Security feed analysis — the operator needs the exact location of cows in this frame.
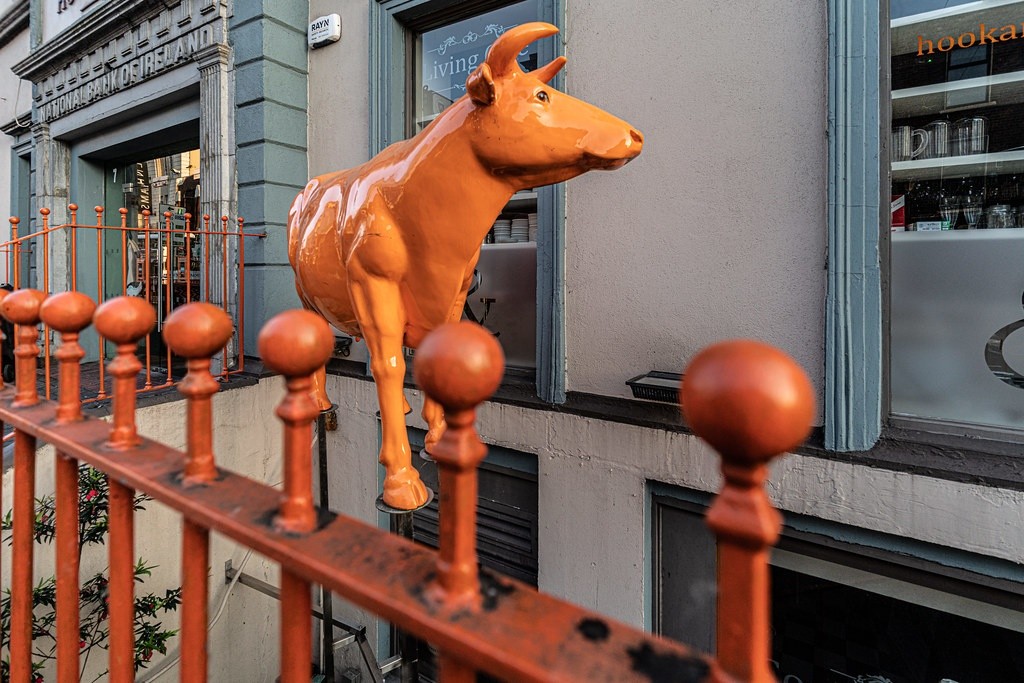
[287,22,645,512]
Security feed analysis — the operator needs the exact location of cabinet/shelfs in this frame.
[417,112,537,250]
[890,0,1024,240]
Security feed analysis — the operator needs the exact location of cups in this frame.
[890,115,985,160]
[899,170,1018,231]
